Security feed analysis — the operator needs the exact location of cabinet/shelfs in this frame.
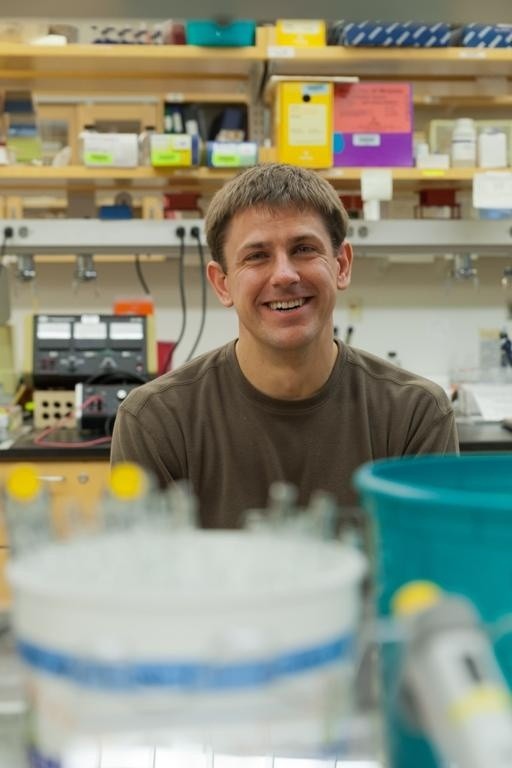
[0,39,511,458]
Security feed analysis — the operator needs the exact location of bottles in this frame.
[479,126,507,167]
[390,579,511,768]
[451,116,476,167]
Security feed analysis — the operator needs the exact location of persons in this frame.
[109,162,460,593]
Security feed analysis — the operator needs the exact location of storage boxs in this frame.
[268,81,335,170]
[78,118,144,167]
[149,133,192,169]
[205,139,259,170]
[184,17,255,44]
[275,17,327,44]
[332,81,414,168]
